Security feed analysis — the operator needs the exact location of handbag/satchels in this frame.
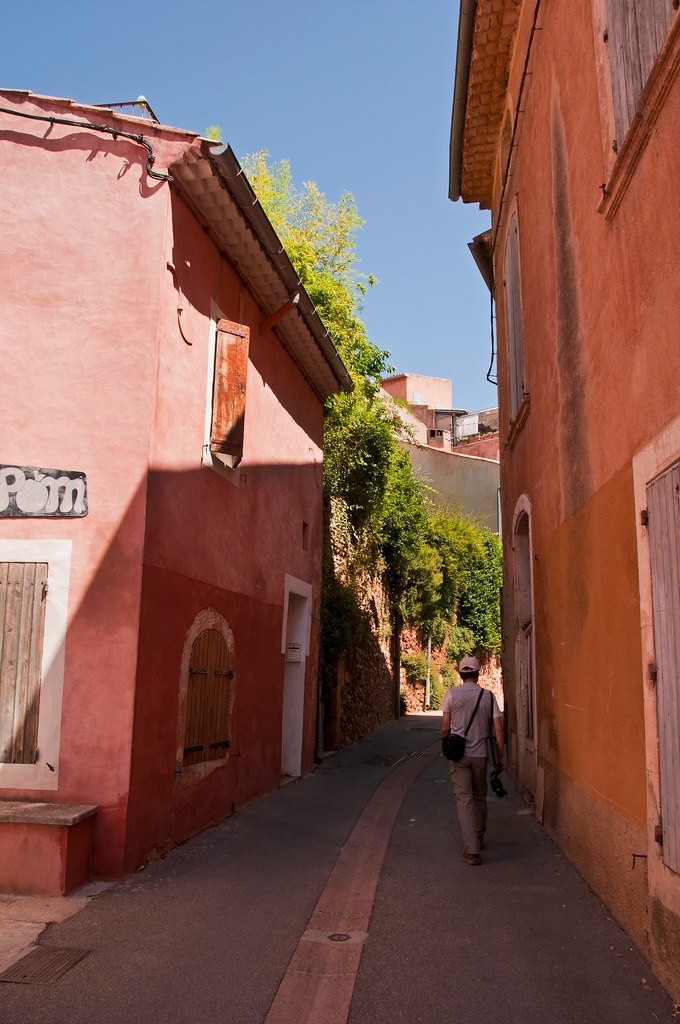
[486,737,499,765]
[441,734,466,760]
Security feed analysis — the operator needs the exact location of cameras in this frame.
[491,779,508,799]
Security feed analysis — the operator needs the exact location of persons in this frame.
[440,656,505,865]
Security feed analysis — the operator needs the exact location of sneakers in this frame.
[462,852,481,865]
[479,838,484,849]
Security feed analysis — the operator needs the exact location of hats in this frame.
[458,657,479,673]
